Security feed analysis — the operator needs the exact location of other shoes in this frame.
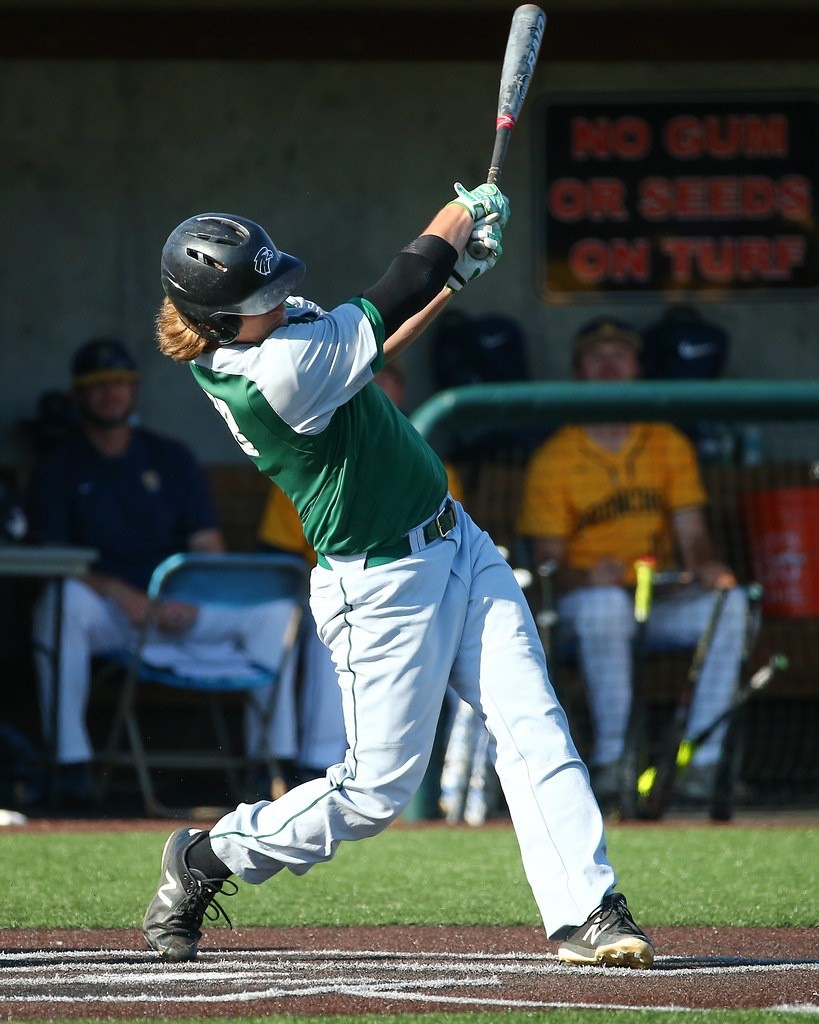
[594,759,636,793]
[609,745,714,758]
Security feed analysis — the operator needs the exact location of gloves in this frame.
[446,181,511,231]
[444,212,503,295]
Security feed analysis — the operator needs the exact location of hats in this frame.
[568,315,641,355]
[70,338,140,387]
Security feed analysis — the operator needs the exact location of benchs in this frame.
[203,464,818,696]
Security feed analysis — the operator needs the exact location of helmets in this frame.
[161,213,307,345]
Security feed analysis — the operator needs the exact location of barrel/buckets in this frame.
[741,488,818,618]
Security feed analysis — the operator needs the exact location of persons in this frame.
[260,362,464,775]
[514,313,747,798]
[22,338,298,815]
[141,183,654,968]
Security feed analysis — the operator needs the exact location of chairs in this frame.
[525,568,762,804]
[88,550,311,818]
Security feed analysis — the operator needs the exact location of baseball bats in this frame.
[464,3,549,263]
[436,548,795,824]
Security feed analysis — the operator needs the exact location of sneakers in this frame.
[140,827,239,962]
[558,892,655,969]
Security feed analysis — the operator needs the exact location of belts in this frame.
[317,500,458,570]
